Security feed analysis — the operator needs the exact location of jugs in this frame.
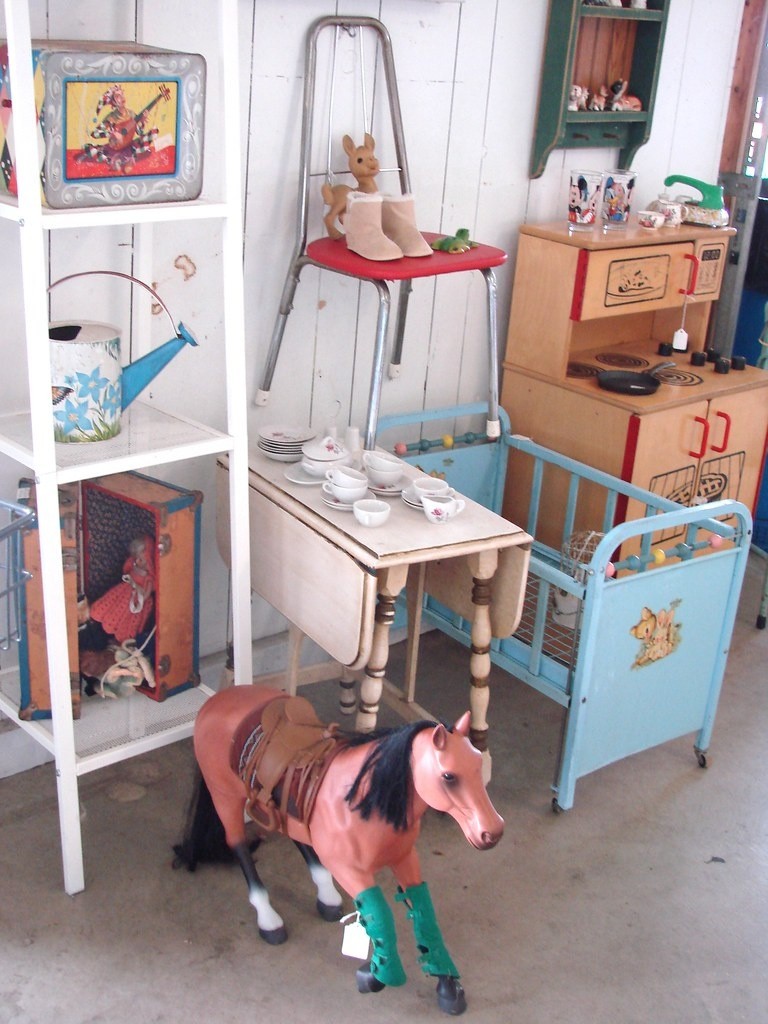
[46,268,201,446]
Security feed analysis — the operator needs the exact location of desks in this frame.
[216,436,533,789]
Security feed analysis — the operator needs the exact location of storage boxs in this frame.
[14,471,204,723]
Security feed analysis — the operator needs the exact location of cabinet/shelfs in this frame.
[0,1,275,896]
[528,1,671,179]
[500,220,767,579]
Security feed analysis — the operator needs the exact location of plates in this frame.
[256,424,316,462]
[284,461,331,486]
[319,490,377,512]
[369,476,413,497]
[400,487,424,511]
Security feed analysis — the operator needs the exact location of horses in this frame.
[168,683,504,1017]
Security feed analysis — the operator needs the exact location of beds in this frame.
[372,401,753,817]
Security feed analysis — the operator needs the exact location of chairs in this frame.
[255,14,508,450]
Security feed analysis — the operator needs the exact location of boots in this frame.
[381,194,434,257]
[345,192,403,260]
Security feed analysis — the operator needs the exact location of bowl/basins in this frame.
[636,210,665,231]
[301,436,353,478]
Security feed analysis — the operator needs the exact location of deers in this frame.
[320,133,383,243]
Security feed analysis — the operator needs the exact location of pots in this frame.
[597,361,677,395]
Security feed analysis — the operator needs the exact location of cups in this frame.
[657,199,689,229]
[412,477,449,503]
[567,169,606,232]
[363,448,404,485]
[603,169,638,230]
[420,494,466,524]
[352,499,391,528]
[322,465,369,504]
